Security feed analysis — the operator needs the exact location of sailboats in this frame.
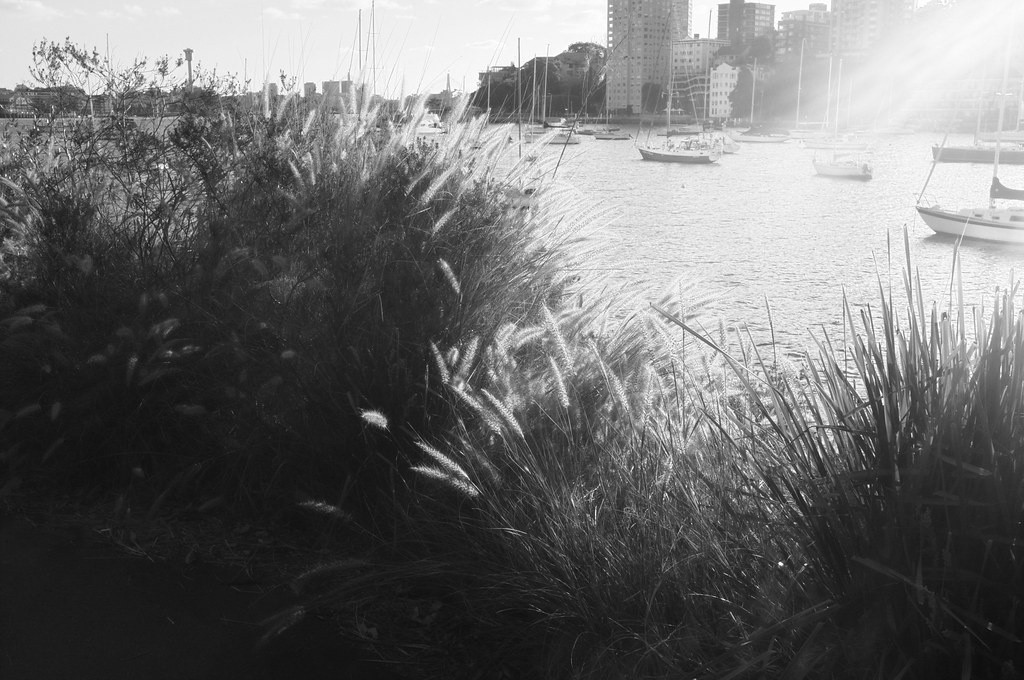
[261,0,1024,246]
